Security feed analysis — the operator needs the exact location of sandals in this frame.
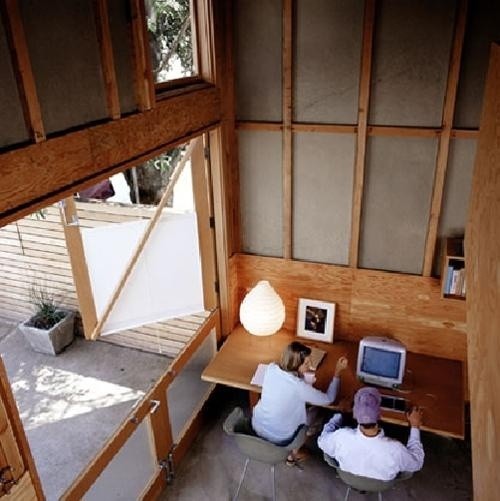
[286,451,309,466]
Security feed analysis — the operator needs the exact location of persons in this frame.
[251,341,348,467]
[318,387,425,493]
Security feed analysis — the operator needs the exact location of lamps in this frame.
[239,280,286,337]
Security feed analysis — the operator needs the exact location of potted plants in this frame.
[18,284,76,358]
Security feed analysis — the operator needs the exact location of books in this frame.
[444,266,466,298]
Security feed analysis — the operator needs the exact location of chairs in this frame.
[323,452,414,501]
[222,406,308,501]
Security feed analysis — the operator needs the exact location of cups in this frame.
[303,372,317,386]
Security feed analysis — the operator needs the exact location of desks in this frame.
[201,322,465,441]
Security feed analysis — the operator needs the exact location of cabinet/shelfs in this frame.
[439,236,466,304]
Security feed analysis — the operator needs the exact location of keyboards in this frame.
[379,394,407,414]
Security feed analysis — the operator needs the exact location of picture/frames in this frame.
[296,298,336,345]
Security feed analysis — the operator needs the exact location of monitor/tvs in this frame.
[356,336,406,390]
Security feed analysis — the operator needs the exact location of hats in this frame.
[352,388,381,424]
